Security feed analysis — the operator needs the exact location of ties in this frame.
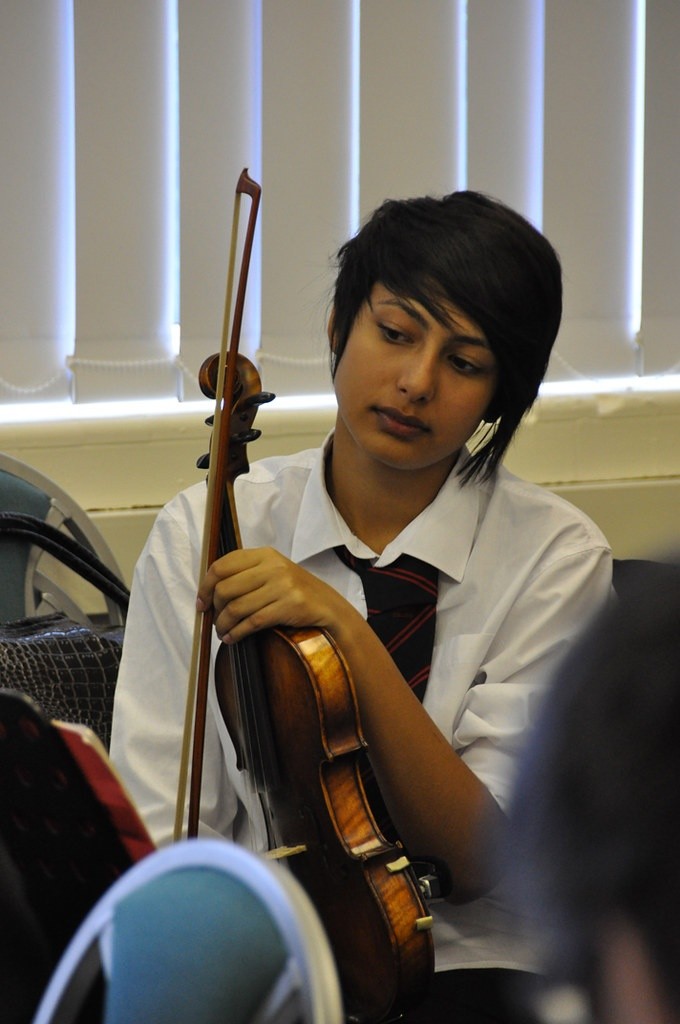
[335,546,438,848]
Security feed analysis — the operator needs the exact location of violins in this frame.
[196,352,435,1024]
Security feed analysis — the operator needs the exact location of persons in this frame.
[461,542,680,1024]
[109,191,612,1024]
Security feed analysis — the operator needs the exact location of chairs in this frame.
[0,454,344,1024]
